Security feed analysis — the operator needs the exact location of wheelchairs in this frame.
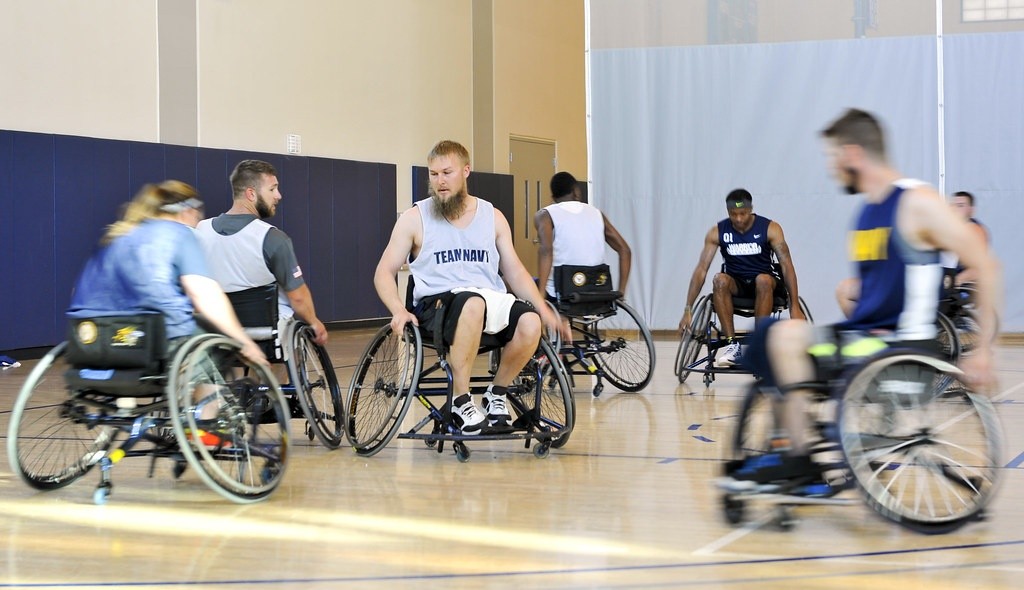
[675,263,815,389]
[343,273,577,465]
[6,310,291,505]
[478,264,657,397]
[189,282,345,449]
[935,278,1003,354]
[722,325,1008,535]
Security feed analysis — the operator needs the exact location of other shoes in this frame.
[182,420,233,448]
[234,395,271,406]
[284,394,299,400]
[537,355,563,364]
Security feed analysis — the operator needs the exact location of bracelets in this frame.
[685,306,691,312]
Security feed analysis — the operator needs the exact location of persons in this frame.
[501,172,631,308]
[374,141,573,434]
[680,189,805,366]
[68,181,271,450]
[196,160,328,411]
[837,193,989,335]
[714,110,1000,499]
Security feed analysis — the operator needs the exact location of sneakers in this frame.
[451,392,492,436]
[480,383,512,426]
[715,342,742,366]
[714,453,833,495]
